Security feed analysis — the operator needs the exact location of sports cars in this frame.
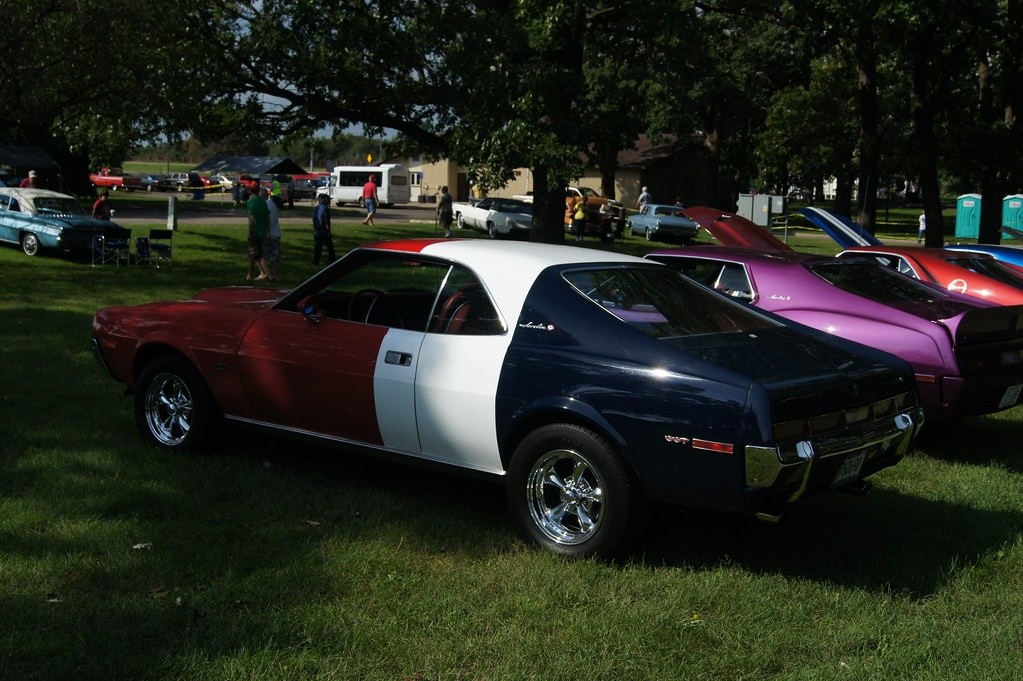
[588,206,1023,436]
[87,236,925,566]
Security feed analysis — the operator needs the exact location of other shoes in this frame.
[255,274,267,281]
[363,222,369,225]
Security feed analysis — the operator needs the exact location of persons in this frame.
[92,189,111,222]
[436,186,454,238]
[20,170,40,188]
[890,180,918,198]
[313,193,336,264]
[918,210,926,244]
[233,176,294,280]
[636,186,652,208]
[362,173,379,227]
[572,195,589,243]
[598,198,614,243]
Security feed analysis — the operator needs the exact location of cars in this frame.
[163,171,326,203]
[133,173,172,190]
[0,186,128,259]
[625,203,699,243]
[449,196,537,238]
[88,167,142,192]
[514,187,626,236]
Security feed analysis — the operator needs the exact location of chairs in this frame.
[366,287,433,329]
[108,228,132,268]
[91,235,118,269]
[144,229,173,266]
[134,236,154,268]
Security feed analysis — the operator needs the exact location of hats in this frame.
[322,194,333,201]
[369,175,376,181]
[29,170,37,177]
[249,181,260,191]
[260,188,271,198]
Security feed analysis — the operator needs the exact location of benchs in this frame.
[431,286,494,334]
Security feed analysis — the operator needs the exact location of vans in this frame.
[315,164,413,208]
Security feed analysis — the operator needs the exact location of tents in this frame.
[190,155,310,176]
